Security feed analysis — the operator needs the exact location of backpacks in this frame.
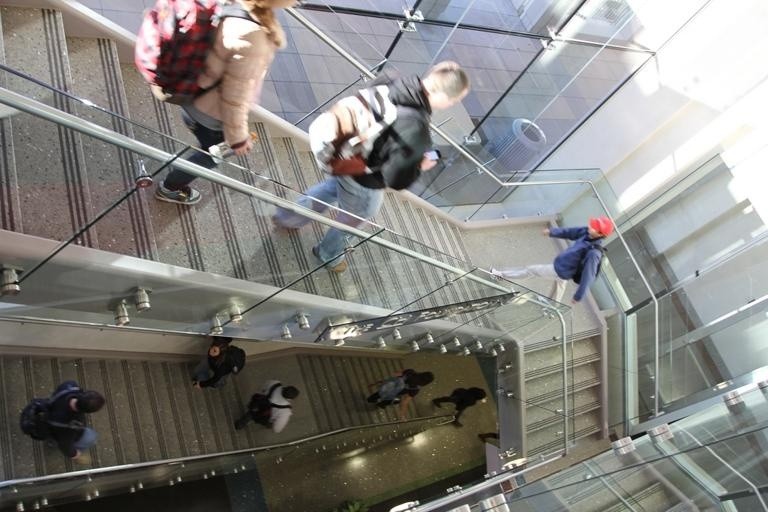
[572,244,608,285]
[307,80,429,177]
[378,371,420,401]
[216,345,246,375]
[248,383,291,425]
[20,385,85,440]
[133,0,262,105]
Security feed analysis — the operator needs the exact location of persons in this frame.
[133,1,295,205]
[189,335,245,389]
[432,386,487,428]
[366,368,434,418]
[21,381,106,459]
[234,379,302,434]
[267,60,470,276]
[489,217,615,310]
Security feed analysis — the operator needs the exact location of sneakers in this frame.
[312,243,349,273]
[432,398,442,408]
[451,420,463,428]
[490,267,505,281]
[154,180,203,205]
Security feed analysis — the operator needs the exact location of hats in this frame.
[589,213,614,239]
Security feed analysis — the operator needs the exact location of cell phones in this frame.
[423,149,442,161]
